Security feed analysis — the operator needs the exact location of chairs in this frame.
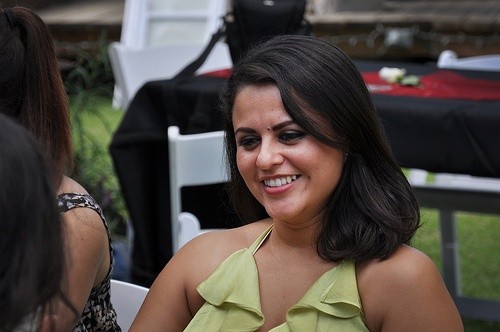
[167,126,255,264]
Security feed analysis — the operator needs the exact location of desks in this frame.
[110,56,500,312]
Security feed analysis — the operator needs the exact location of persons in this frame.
[1,115,69,331]
[124,35,466,332]
[1,8,119,331]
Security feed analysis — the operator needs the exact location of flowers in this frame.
[378,67,425,90]
